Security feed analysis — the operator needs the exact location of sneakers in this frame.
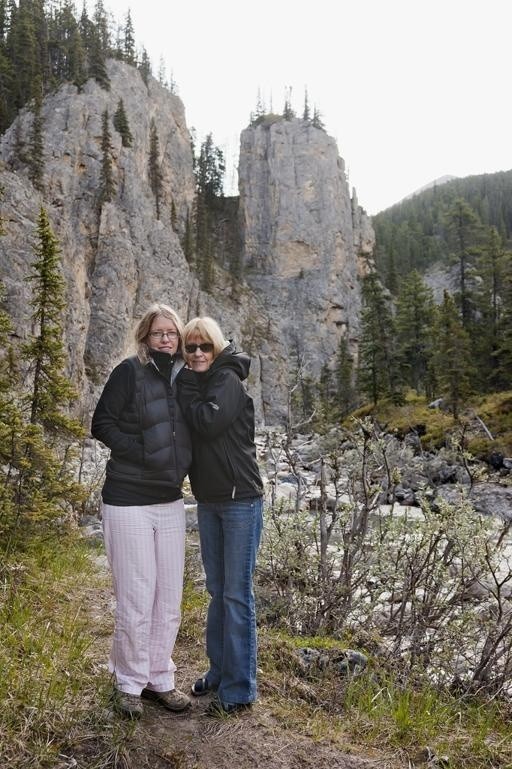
[110,687,142,719]
[191,671,252,718]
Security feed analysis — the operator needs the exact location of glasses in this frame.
[149,330,177,339]
[185,343,214,353]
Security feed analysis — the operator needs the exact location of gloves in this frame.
[143,686,192,713]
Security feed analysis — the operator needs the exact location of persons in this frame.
[91,303,193,715]
[176,316,266,716]
